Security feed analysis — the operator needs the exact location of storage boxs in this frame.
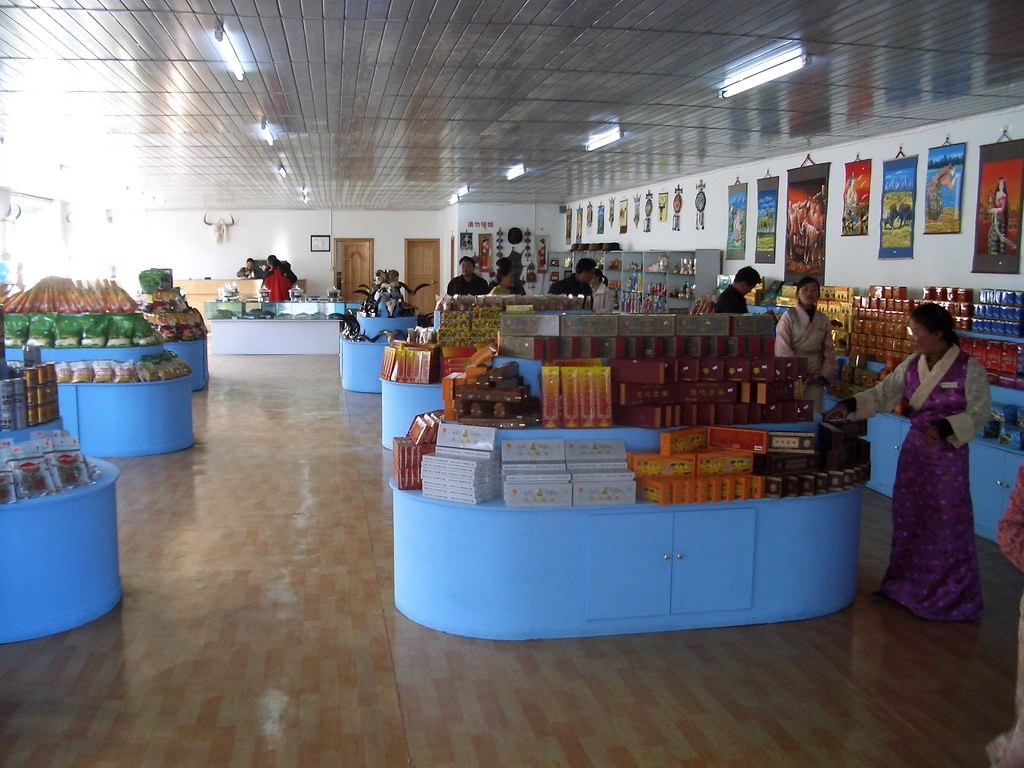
[380,259,1024,506]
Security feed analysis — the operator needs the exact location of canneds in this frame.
[0,345,59,432]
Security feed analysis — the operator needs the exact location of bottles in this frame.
[620,258,669,313]
[671,257,696,300]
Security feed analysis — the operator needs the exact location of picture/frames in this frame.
[311,235,330,252]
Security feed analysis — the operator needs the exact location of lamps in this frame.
[214,31,244,82]
[302,194,308,205]
[449,196,460,205]
[718,53,814,99]
[261,122,274,147]
[278,166,287,179]
[507,168,528,182]
[585,132,625,152]
[459,187,470,197]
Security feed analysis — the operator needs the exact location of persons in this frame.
[261,255,297,285]
[447,256,490,295]
[489,258,526,296]
[237,258,264,279]
[261,262,291,303]
[488,268,516,295]
[774,275,837,413]
[822,302,992,622]
[712,266,761,313]
[589,269,613,313]
[547,257,596,309]
[987,462,1024,768]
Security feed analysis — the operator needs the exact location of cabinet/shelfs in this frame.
[0,416,121,644]
[377,310,446,451]
[137,308,210,391]
[389,358,862,641]
[747,304,1024,544]
[337,312,417,395]
[4,312,195,459]
[544,249,721,314]
